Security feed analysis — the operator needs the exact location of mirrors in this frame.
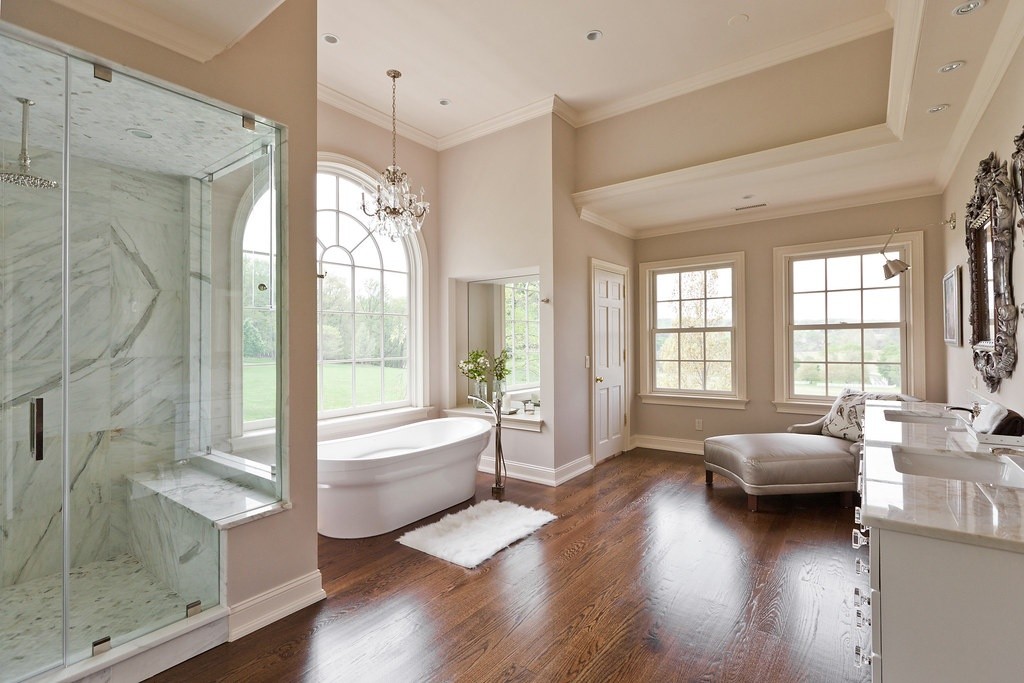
[963,151,1019,394]
[466,272,541,412]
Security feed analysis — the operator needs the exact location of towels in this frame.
[971,401,1024,437]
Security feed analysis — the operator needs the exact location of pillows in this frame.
[821,386,909,442]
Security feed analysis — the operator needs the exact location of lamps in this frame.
[880,211,957,280]
[360,166,430,242]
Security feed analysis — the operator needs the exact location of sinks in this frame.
[883,408,968,427]
[890,443,1024,489]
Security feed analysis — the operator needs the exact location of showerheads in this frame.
[1,96,59,191]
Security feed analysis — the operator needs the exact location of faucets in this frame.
[467,393,500,425]
[943,402,982,426]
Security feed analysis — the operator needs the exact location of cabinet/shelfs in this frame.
[852,447,1022,682]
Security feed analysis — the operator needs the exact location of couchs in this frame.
[701,388,925,511]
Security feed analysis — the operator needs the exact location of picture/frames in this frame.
[942,265,962,347]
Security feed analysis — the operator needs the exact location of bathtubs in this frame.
[317,416,493,539]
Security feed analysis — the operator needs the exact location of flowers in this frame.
[490,349,514,380]
[459,349,490,379]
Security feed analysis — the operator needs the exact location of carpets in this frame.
[394,498,558,570]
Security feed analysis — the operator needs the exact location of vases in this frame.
[472,382,487,408]
[492,380,508,411]
